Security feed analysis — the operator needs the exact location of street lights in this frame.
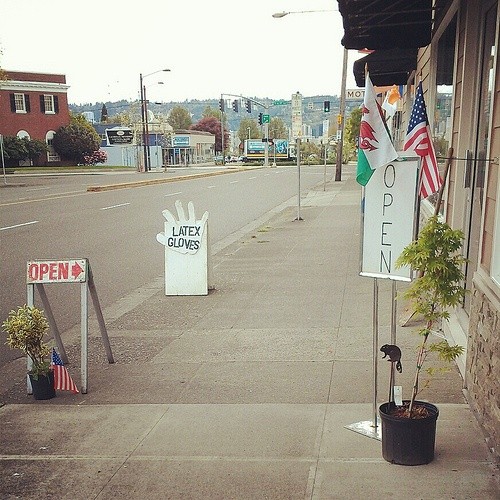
[140,69,172,172]
[143,81,165,171]
[272,9,349,182]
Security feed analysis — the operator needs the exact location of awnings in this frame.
[337,0,432,87]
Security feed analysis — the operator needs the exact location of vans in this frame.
[214,156,245,162]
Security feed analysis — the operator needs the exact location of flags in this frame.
[403,82,443,201]
[356,70,398,186]
[52,348,79,395]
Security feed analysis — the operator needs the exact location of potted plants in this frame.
[379,214,474,466]
[2,303,55,400]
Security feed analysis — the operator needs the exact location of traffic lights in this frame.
[219,99,225,112]
[245,100,251,113]
[324,101,330,112]
[232,99,238,113]
[258,113,263,126]
